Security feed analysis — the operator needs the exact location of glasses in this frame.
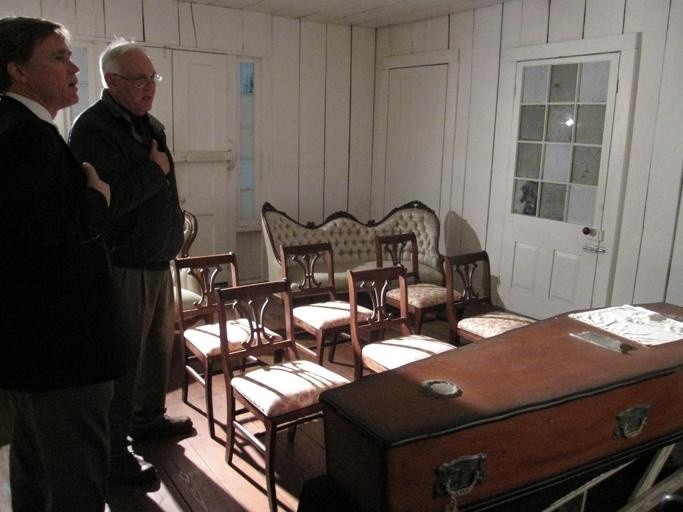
[112,73,158,90]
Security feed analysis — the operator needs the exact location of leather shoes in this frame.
[132,410,193,443]
[110,446,158,489]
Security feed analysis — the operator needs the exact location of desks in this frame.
[320,303,683,512]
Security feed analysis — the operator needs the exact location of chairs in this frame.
[172,251,285,439]
[374,234,466,335]
[279,241,379,368]
[436,249,537,349]
[346,263,458,382]
[214,277,351,511]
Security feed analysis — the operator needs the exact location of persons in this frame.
[1,13,130,512]
[58,30,195,484]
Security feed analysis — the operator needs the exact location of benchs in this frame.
[261,202,445,304]
[170,209,214,331]
